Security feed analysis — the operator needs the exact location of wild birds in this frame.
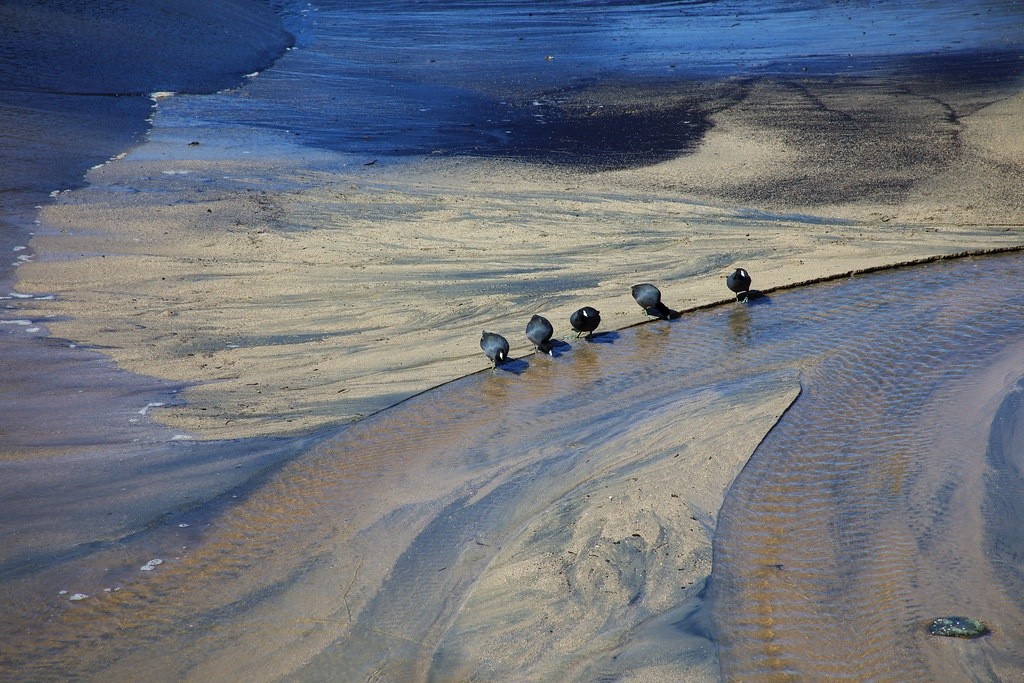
[726,267,752,304]
[630,283,672,321]
[479,329,510,372]
[569,306,601,343]
[525,314,554,357]
